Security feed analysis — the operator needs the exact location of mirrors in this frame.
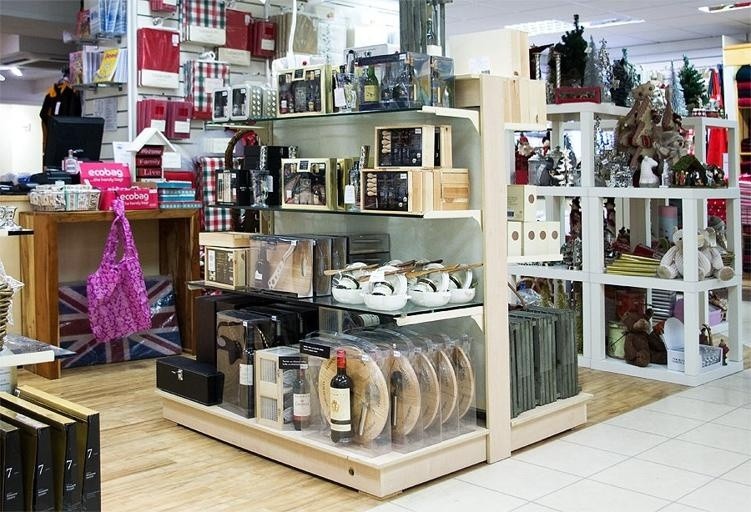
[1,0,138,189]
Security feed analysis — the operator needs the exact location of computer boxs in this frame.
[30,172,80,185]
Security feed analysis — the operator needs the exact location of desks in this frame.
[0,350,55,369]
[18,211,198,379]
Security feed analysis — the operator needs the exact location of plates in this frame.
[318,345,475,446]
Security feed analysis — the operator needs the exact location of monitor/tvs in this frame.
[44,116,105,169]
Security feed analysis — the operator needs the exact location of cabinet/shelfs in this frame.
[505,104,744,386]
[155,72,498,499]
[498,72,593,463]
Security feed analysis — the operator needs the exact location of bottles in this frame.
[255,247,270,288]
[292,369,310,431]
[280,83,321,114]
[64,149,78,175]
[363,62,441,109]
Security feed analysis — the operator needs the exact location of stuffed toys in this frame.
[606,288,684,367]
[656,215,735,281]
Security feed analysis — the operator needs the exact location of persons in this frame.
[602,198,631,267]
[515,130,582,188]
[699,328,708,344]
[559,197,582,270]
[719,339,729,365]
